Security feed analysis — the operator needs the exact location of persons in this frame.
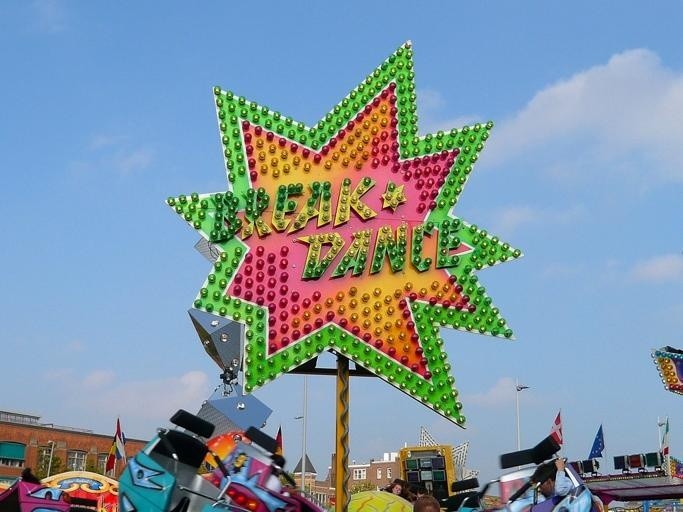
[410,488,428,506]
[521,459,576,512]
[390,483,403,495]
[413,494,440,512]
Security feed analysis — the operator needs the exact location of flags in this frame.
[114,419,125,460]
[587,424,604,459]
[551,412,562,445]
[657,416,671,448]
[105,438,116,474]
